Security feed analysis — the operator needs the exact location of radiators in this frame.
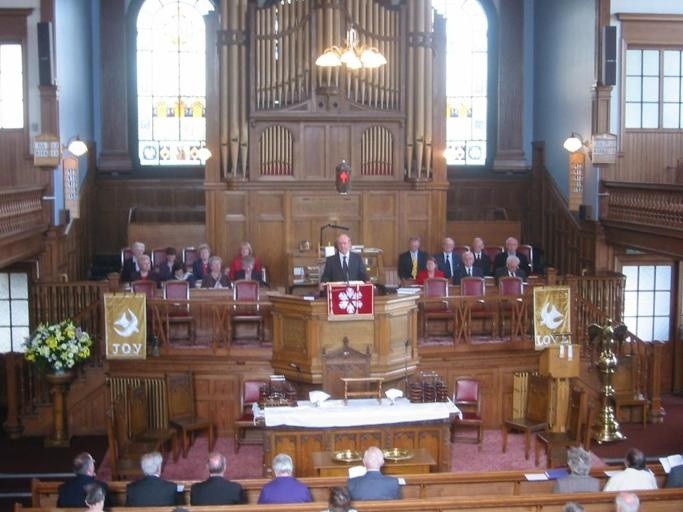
[104,376,168,438]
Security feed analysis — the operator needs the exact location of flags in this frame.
[533,286,573,351]
[326,283,375,321]
[103,290,148,362]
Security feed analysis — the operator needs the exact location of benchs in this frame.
[12,460,682,511]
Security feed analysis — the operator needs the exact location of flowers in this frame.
[19,318,94,371]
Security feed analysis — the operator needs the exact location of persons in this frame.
[319,233,374,293]
[322,484,358,512]
[233,255,264,283]
[56,450,111,509]
[452,250,485,283]
[226,240,264,279]
[493,235,529,275]
[470,235,492,276]
[397,236,429,282]
[603,444,660,492]
[168,258,197,287]
[415,255,446,284]
[125,449,183,508]
[613,491,644,512]
[493,253,528,286]
[551,445,602,493]
[119,241,154,279]
[256,452,315,505]
[188,449,243,507]
[82,481,108,512]
[127,254,161,286]
[200,254,230,288]
[192,242,213,277]
[346,444,403,500]
[154,246,187,278]
[434,235,463,278]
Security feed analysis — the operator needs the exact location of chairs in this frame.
[159,279,196,347]
[606,352,651,433]
[150,248,168,272]
[459,276,501,344]
[228,278,264,344]
[501,373,548,461]
[119,246,134,269]
[449,376,484,444]
[338,376,385,399]
[420,277,457,342]
[451,244,472,257]
[129,279,157,321]
[515,244,534,275]
[482,244,505,273]
[496,275,524,313]
[164,368,214,459]
[231,375,268,455]
[181,245,200,267]
[533,383,587,470]
[124,380,179,460]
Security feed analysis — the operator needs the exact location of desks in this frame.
[536,343,582,433]
[249,395,462,477]
[264,292,421,384]
[286,245,386,292]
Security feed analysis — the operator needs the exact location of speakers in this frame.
[38,22,54,86]
[601,26,616,85]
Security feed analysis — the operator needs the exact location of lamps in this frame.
[313,25,389,72]
[560,129,591,154]
[61,131,90,158]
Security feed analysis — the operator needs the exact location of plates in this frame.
[381,448,415,464]
[330,449,364,463]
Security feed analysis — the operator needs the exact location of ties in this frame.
[342,256,348,271]
[510,272,515,278]
[476,255,481,263]
[468,269,471,277]
[411,255,418,279]
[444,255,452,278]
